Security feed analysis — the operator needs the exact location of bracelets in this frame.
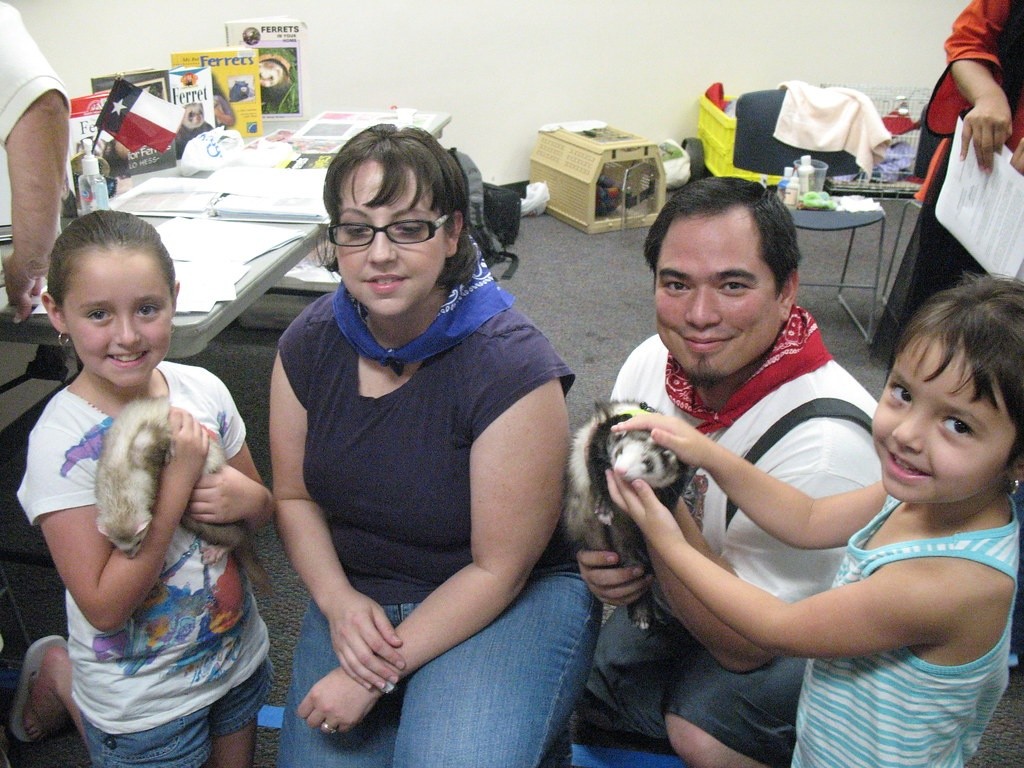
[380,681,395,693]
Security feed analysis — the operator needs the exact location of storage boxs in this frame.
[697,93,783,186]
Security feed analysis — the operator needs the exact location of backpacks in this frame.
[446,146,519,280]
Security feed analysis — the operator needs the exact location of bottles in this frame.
[798,155,816,194]
[783,176,800,206]
[776,167,794,201]
[78,139,109,215]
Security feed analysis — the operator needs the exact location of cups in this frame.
[794,159,829,194]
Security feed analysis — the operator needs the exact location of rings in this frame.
[321,722,336,733]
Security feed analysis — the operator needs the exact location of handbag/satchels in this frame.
[175,126,244,177]
[481,181,521,247]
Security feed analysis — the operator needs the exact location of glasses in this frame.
[329,215,451,247]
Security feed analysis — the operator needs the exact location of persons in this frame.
[267,124,605,768]
[12,211,273,768]
[1,0,72,324]
[580,0,1024,768]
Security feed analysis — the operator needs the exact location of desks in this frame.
[785,195,886,343]
[0,109,451,393]
[882,199,923,305]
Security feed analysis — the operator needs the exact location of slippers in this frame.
[9,635,71,743]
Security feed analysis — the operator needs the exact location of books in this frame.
[68,20,418,225]
[935,115,1023,281]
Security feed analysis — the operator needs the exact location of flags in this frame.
[96,72,185,154]
[103,130,145,153]
